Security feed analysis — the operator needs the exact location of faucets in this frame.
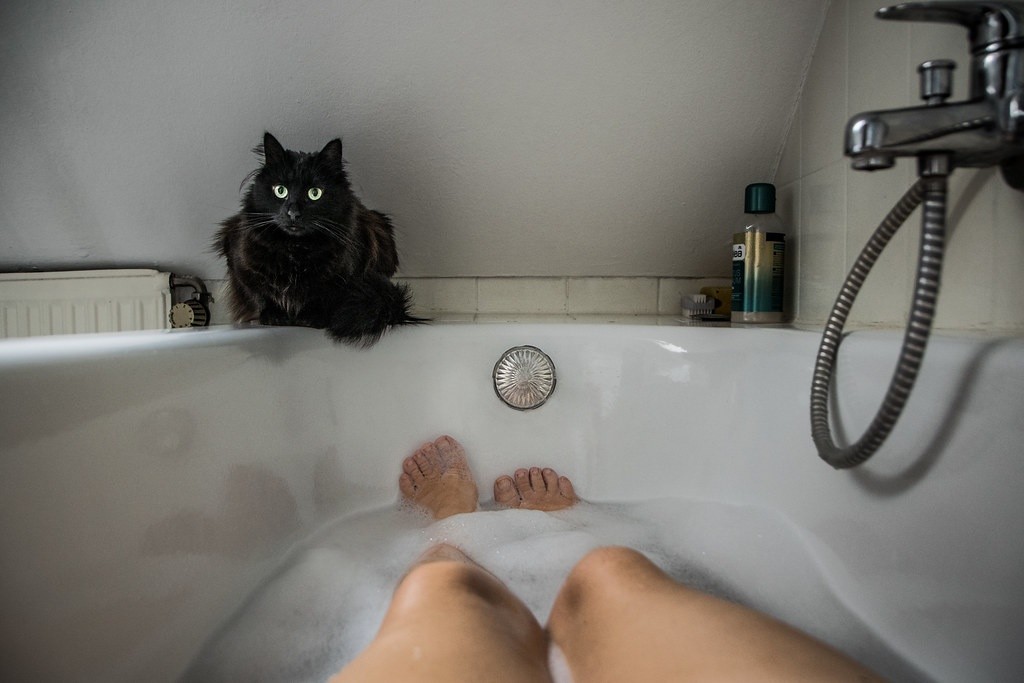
[842,0,1023,179]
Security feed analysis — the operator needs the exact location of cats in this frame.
[193,129,436,350]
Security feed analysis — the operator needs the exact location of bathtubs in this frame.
[0,321,1022,682]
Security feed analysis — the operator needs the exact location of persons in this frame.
[329,434,896,683]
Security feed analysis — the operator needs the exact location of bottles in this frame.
[730,182,788,323]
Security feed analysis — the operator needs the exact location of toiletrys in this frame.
[729,182,785,326]
